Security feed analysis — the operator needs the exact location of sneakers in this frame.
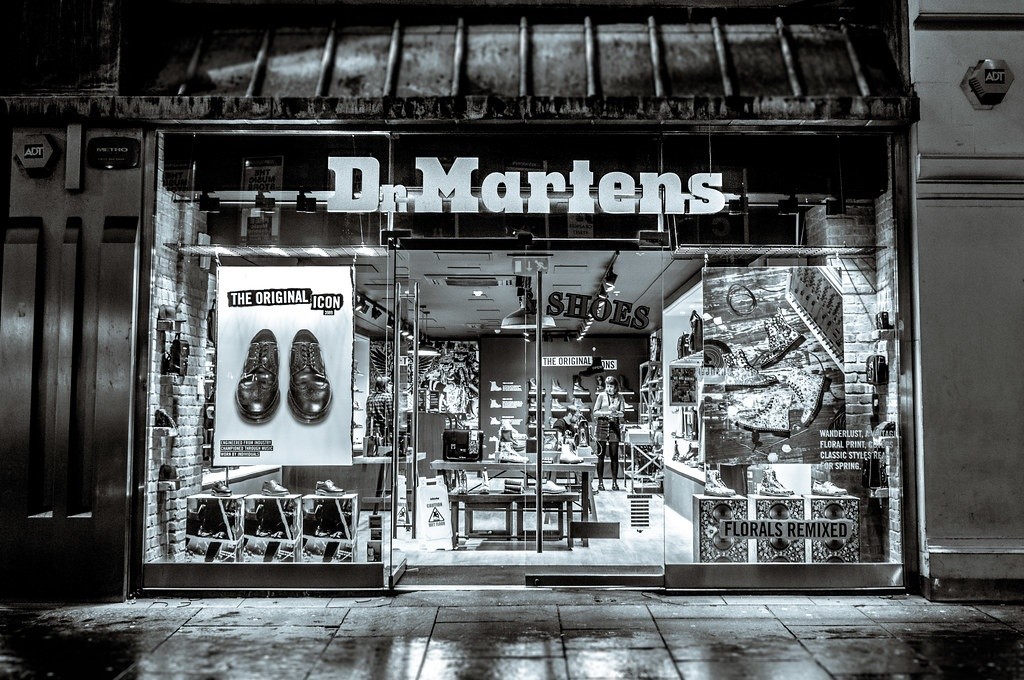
[533,481,566,493]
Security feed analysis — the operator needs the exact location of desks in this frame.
[430,460,596,548]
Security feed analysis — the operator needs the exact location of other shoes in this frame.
[612,483,619,491]
[598,483,605,492]
[156,408,176,428]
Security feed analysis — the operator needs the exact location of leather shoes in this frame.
[235,325,333,429]
[315,479,346,497]
[211,482,233,497]
[261,480,289,496]
[813,480,847,496]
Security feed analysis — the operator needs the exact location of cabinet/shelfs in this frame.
[186,332,442,568]
[623,272,705,521]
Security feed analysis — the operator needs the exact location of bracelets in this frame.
[601,412,605,417]
[616,413,619,416]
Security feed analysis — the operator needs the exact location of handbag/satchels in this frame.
[442,429,484,462]
[876,312,889,329]
[866,354,886,384]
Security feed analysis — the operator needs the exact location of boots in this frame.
[500,430,525,452]
[573,382,591,396]
[529,378,546,394]
[498,442,529,463]
[722,350,778,393]
[760,311,806,369]
[528,398,545,412]
[551,378,567,393]
[703,470,736,496]
[785,367,833,427]
[574,398,591,411]
[528,414,545,428]
[735,391,792,437]
[560,444,585,464]
[552,398,567,411]
[760,469,795,497]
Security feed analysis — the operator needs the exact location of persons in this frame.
[593,376,625,491]
[365,376,393,436]
[553,405,579,437]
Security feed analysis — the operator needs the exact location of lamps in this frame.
[353,291,425,344]
[412,311,441,357]
[576,269,617,341]
[500,277,556,330]
[407,305,438,355]
